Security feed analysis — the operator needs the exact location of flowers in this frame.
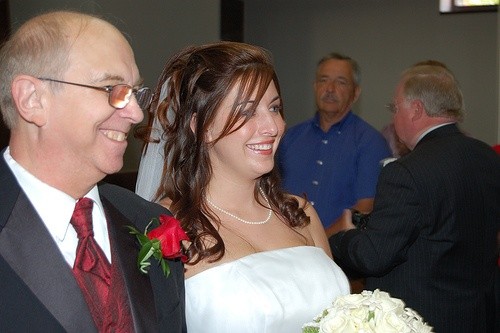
[299,287,437,333]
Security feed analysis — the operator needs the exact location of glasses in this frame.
[34,74,155,111]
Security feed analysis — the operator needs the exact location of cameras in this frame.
[344,208,373,229]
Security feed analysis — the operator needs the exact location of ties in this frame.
[71,197,112,332]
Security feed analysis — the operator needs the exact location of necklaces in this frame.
[211,184,273,225]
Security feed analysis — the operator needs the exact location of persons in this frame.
[134,39,352,333]
[0,11,185,332]
[273,51,394,238]
[342,60,500,333]
